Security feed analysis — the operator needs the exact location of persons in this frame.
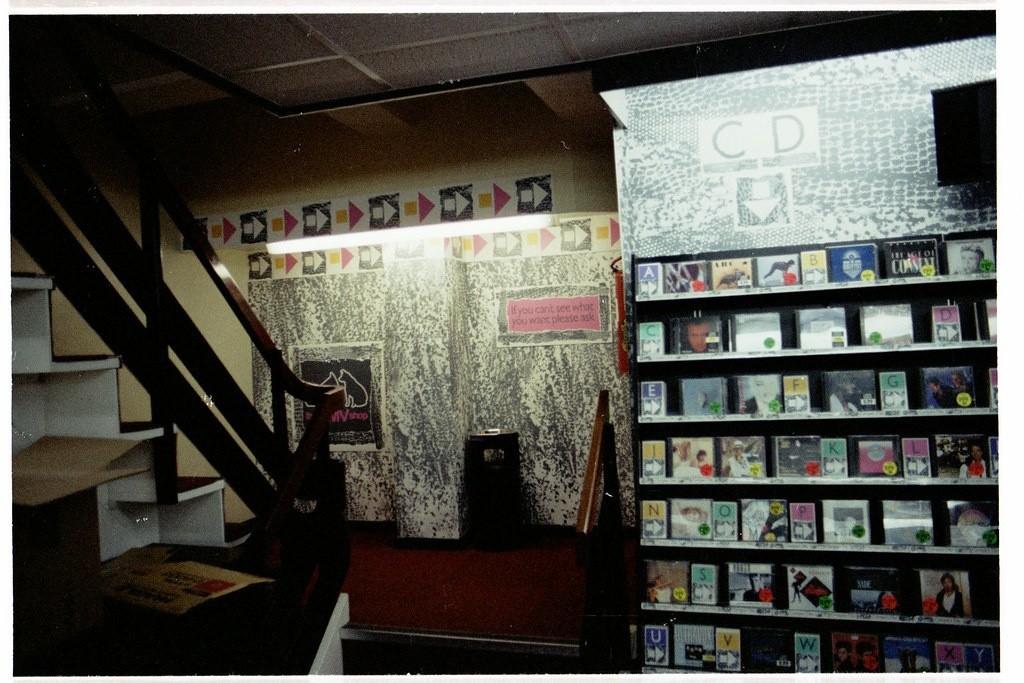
[729,440,751,477]
[683,318,713,353]
[927,370,974,408]
[742,573,768,602]
[764,260,794,278]
[935,573,964,617]
[937,436,987,477]
[680,506,708,522]
[954,245,984,275]
